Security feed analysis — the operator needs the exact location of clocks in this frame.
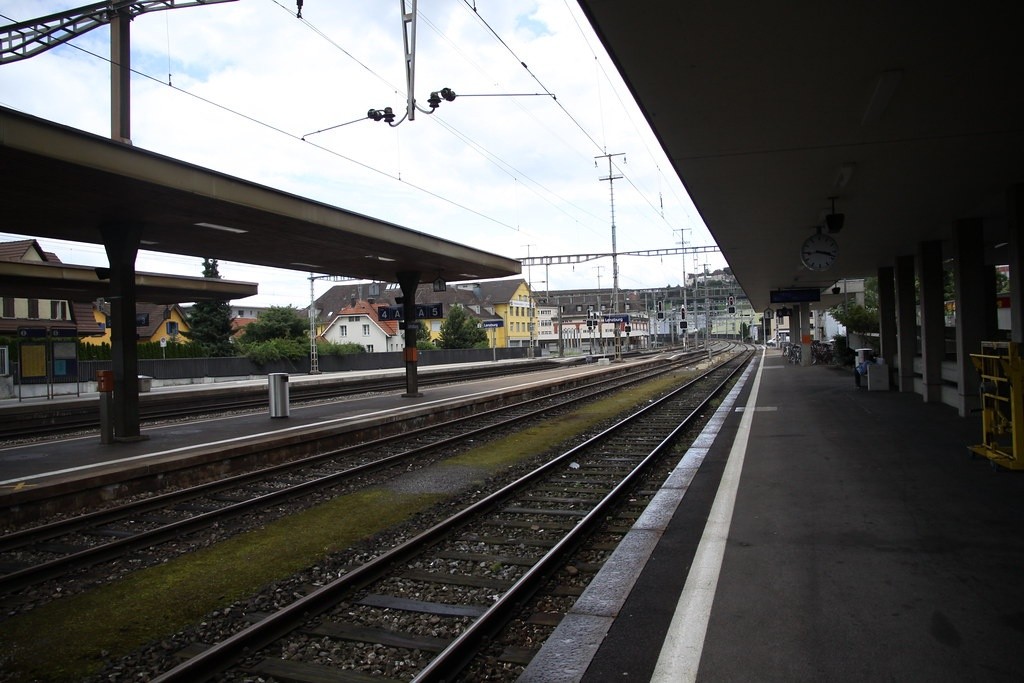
[800,226,840,272]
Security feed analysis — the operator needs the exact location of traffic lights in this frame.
[587,309,590,319]
[594,312,597,319]
[681,308,685,319]
[587,320,597,326]
[657,301,661,312]
[729,296,734,306]
[729,307,735,313]
[680,322,687,328]
[625,326,631,332]
[658,313,663,318]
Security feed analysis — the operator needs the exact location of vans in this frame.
[766,336,790,346]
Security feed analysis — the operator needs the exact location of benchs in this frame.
[860,357,889,391]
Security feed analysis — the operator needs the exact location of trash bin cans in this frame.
[268,373,289,419]
[854,348,872,387]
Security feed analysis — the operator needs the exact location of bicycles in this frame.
[782,340,833,367]
